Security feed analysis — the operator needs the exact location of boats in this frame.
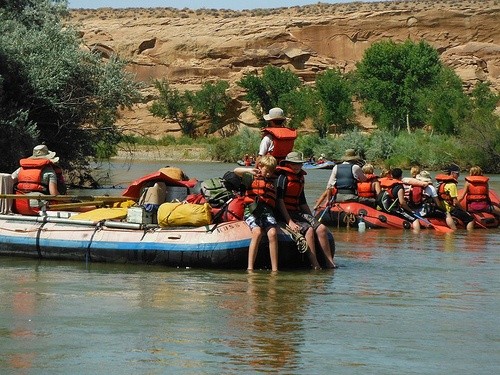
[313,187,500,232]
[236,159,256,166]
[0,166,337,271]
[302,160,336,169]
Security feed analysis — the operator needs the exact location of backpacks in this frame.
[377,190,399,213]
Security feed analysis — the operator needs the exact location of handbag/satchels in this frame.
[157,171,247,227]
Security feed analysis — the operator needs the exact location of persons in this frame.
[233,107,336,276]
[312,149,500,235]
[10,144,68,216]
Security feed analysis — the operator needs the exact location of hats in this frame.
[448,163,460,172]
[279,152,306,166]
[318,157,323,160]
[50,157,59,164]
[28,145,56,159]
[321,154,325,157]
[341,149,360,162]
[416,171,432,181]
[244,154,249,157]
[263,108,289,121]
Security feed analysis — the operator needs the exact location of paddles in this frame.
[318,194,335,222]
[458,205,488,229]
[413,213,453,232]
[0,193,138,202]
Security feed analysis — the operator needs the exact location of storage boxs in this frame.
[144,186,188,202]
[127,207,157,224]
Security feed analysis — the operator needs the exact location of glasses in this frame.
[456,173,459,176]
